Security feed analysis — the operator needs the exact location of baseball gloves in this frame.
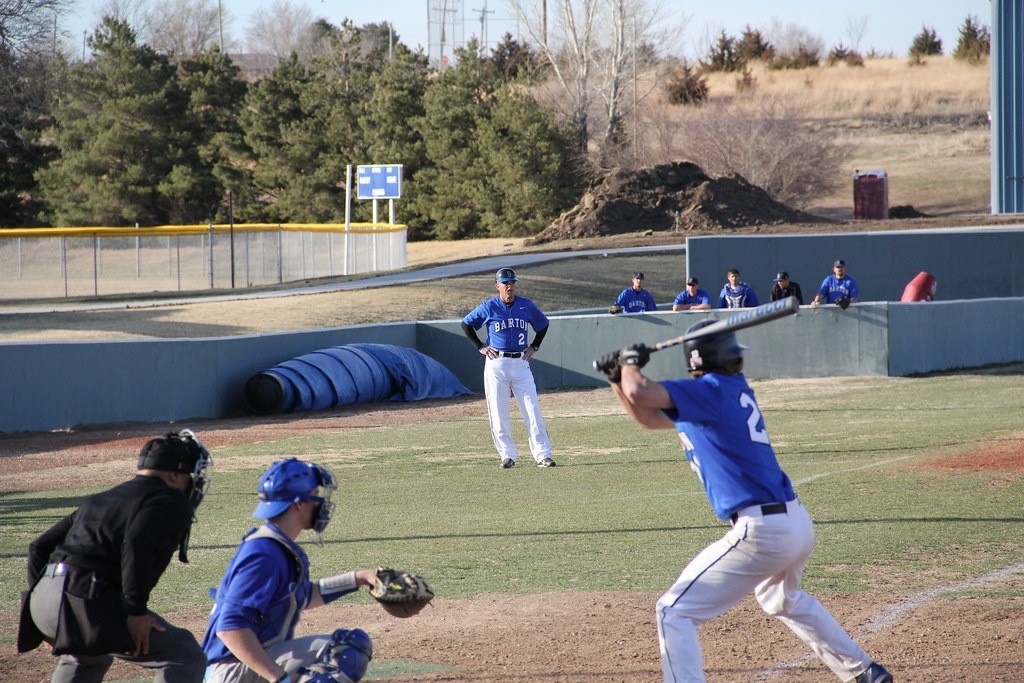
[836,295,850,310]
[369,566,433,620]
[609,306,625,314]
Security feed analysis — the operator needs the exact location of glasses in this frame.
[502,281,516,285]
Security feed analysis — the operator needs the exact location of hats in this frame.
[687,276,698,285]
[834,260,846,267]
[773,272,789,282]
[137,439,208,474]
[632,271,644,279]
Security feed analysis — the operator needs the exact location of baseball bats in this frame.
[591,296,799,372]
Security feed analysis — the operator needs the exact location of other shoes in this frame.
[538,458,556,468]
[855,664,893,683]
[499,458,515,468]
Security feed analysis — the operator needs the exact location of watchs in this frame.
[530,344,538,351]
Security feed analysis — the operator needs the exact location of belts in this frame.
[730,502,787,521]
[495,351,521,359]
[43,562,68,575]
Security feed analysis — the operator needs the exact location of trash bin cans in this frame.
[853,170,888,219]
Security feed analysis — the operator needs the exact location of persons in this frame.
[717,269,760,309]
[599,318,893,683]
[16,430,213,683]
[203,458,433,683]
[673,277,713,311]
[811,260,858,308]
[461,268,556,468]
[769,272,803,304]
[608,272,658,313]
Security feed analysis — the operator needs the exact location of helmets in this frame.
[495,268,520,283]
[249,457,321,518]
[683,319,750,370]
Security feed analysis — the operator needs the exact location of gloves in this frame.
[600,350,621,382]
[618,343,650,368]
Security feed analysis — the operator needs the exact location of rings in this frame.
[528,354,530,356]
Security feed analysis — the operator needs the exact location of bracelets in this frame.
[317,570,359,606]
